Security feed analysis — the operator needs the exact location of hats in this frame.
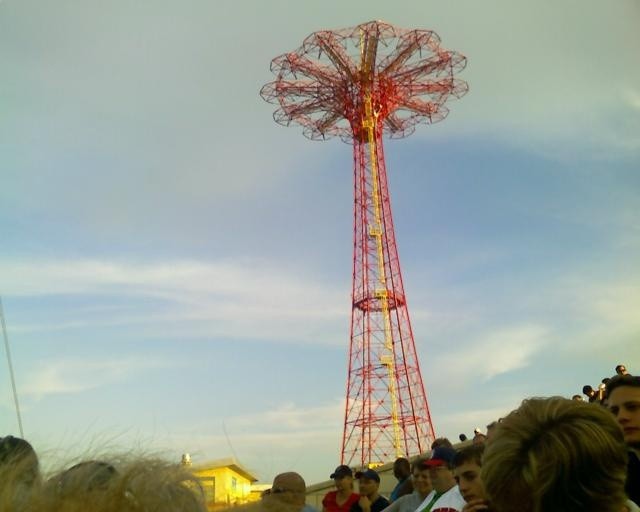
[330,464,353,479]
[355,467,380,483]
[422,445,456,469]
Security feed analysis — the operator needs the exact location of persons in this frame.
[384,365,639,511]
[0,435,208,512]
[260,465,389,512]
